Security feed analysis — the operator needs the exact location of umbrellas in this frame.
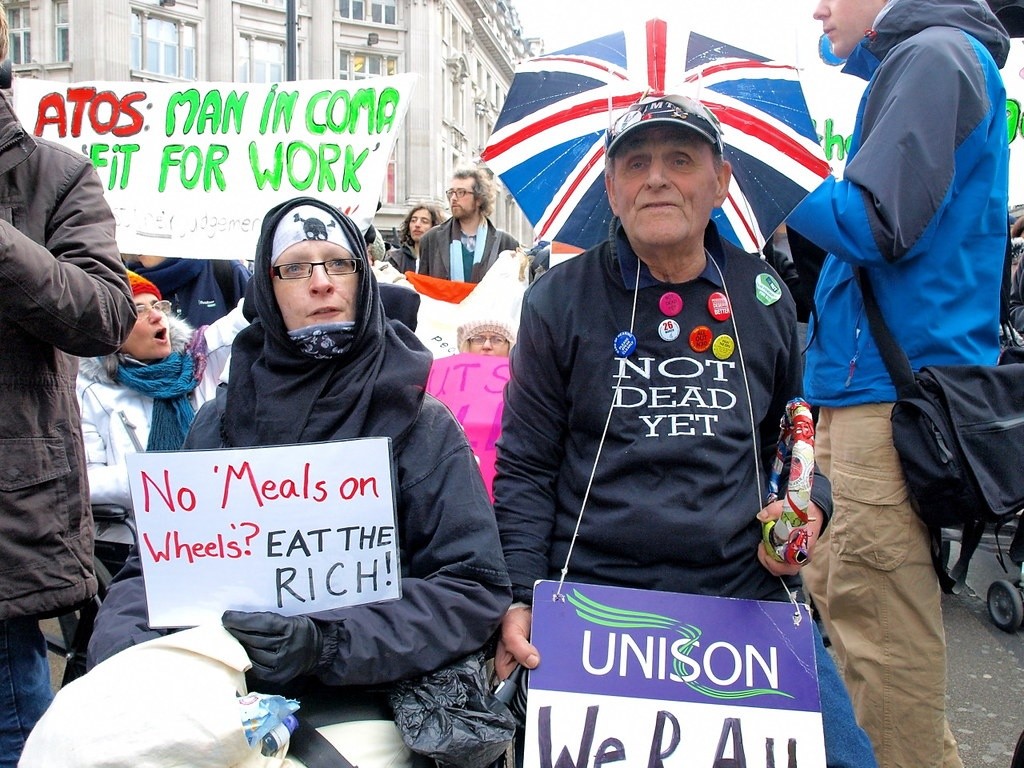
[478,17,840,261]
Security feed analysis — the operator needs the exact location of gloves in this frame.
[221,610,338,683]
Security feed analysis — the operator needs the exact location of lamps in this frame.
[367,33,379,47]
[159,0,176,7]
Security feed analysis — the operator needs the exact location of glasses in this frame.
[468,335,507,346]
[136,300,172,320]
[271,258,362,280]
[445,188,474,198]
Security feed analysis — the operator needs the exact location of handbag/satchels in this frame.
[892,345,1023,529]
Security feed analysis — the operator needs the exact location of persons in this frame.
[0,0,1023,767]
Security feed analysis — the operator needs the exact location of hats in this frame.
[124,267,161,301]
[271,205,357,266]
[605,91,724,152]
[456,319,516,355]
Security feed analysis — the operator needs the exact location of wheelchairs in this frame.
[54,503,140,677]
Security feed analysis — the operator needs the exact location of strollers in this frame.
[985,348,1024,632]
